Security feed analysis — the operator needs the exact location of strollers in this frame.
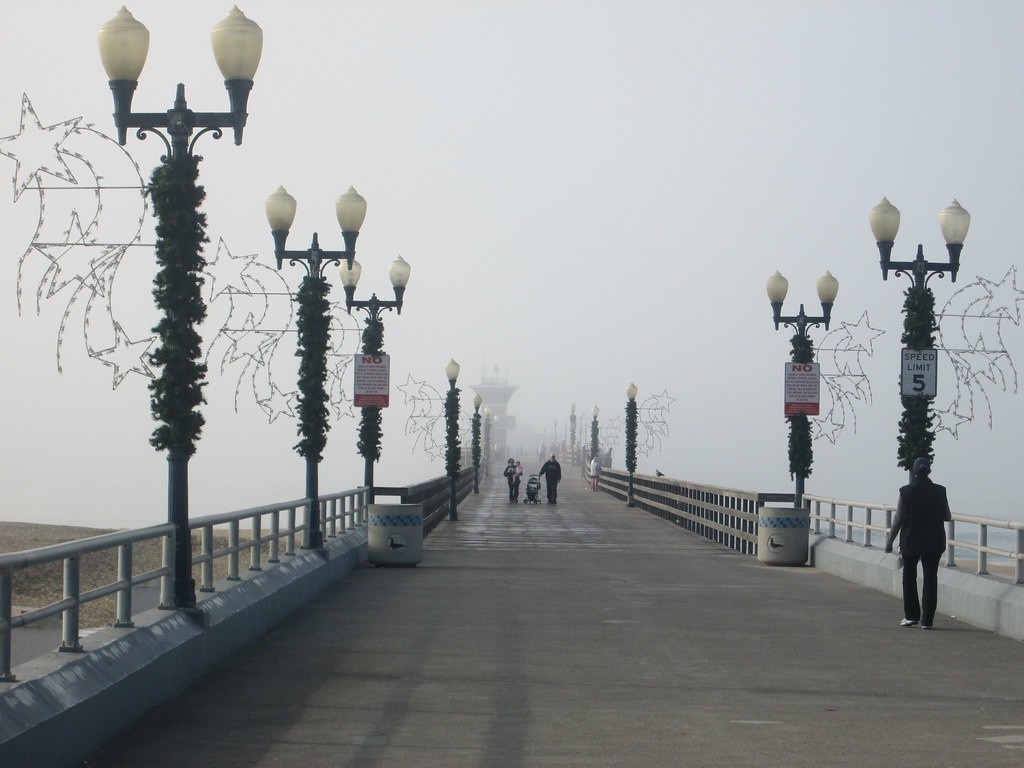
[522,474,541,505]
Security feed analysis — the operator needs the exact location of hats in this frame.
[914,457,930,471]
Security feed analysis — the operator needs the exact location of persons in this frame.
[539,454,561,504]
[539,440,567,466]
[590,454,600,492]
[504,458,522,503]
[885,457,951,629]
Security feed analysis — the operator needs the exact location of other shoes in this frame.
[513,497,518,503]
[923,623,933,629]
[510,497,513,503]
[900,618,918,626]
[547,498,557,504]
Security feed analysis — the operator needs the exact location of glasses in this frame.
[510,461,514,464]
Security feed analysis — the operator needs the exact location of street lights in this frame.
[567,402,578,465]
[473,393,484,494]
[553,418,559,446]
[588,403,600,463]
[338,254,413,523]
[868,194,971,484]
[262,184,370,552]
[766,270,839,506]
[97,0,266,615]
[441,358,462,521]
[624,381,639,508]
[484,404,494,477]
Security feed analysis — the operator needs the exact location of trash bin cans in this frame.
[367,504,422,566]
[758,506,810,568]
